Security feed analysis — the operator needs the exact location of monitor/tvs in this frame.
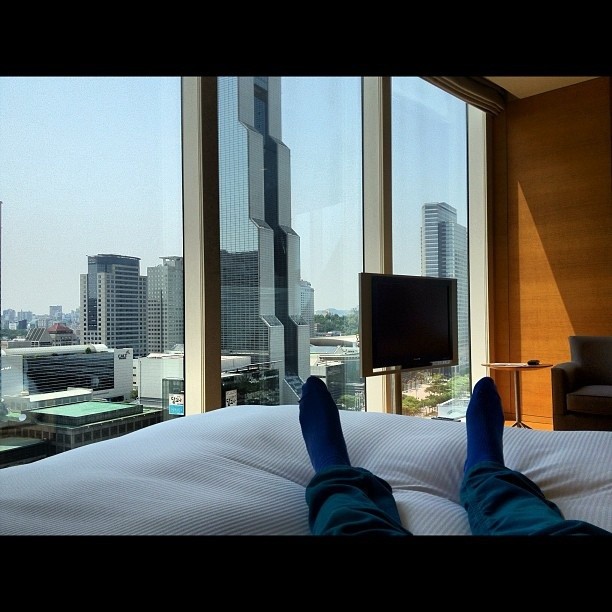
[357,271,459,379]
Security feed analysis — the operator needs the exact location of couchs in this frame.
[551,336,611,431]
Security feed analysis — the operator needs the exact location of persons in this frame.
[299,376,612,537]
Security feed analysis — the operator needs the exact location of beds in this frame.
[0,405,611,611]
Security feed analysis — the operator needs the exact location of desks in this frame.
[484,361,554,428]
